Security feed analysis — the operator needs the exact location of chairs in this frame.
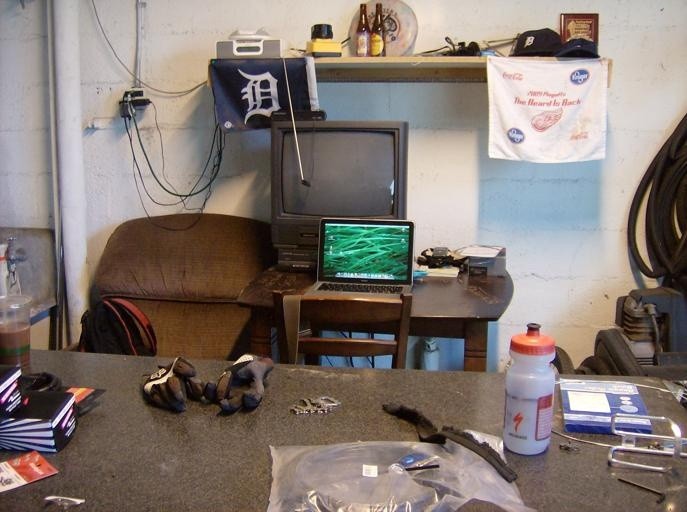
[270,288,413,369]
[63,212,276,362]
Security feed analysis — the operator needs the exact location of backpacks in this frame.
[77,297,158,356]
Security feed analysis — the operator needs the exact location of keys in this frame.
[288,392,344,416]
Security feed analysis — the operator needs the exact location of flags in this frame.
[211,56,320,133]
[484,53,609,163]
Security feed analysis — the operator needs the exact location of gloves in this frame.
[204,353,275,415]
[141,357,204,413]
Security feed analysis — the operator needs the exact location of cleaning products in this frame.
[0,236,31,374]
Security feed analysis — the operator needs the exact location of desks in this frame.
[235,259,515,372]
[0,347,687,512]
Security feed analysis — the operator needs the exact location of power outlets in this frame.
[123,88,146,112]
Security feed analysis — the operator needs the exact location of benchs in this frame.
[0,225,57,351]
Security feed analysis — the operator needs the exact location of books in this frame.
[418,263,460,278]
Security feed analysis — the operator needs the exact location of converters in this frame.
[129,98,150,107]
[123,90,143,98]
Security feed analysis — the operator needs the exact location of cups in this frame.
[0,294,33,372]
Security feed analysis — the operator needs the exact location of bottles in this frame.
[355,4,371,57]
[371,3,386,57]
[503,322,556,456]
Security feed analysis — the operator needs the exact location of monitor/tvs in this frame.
[269,118,409,245]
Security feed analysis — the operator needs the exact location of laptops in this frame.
[304,217,416,295]
